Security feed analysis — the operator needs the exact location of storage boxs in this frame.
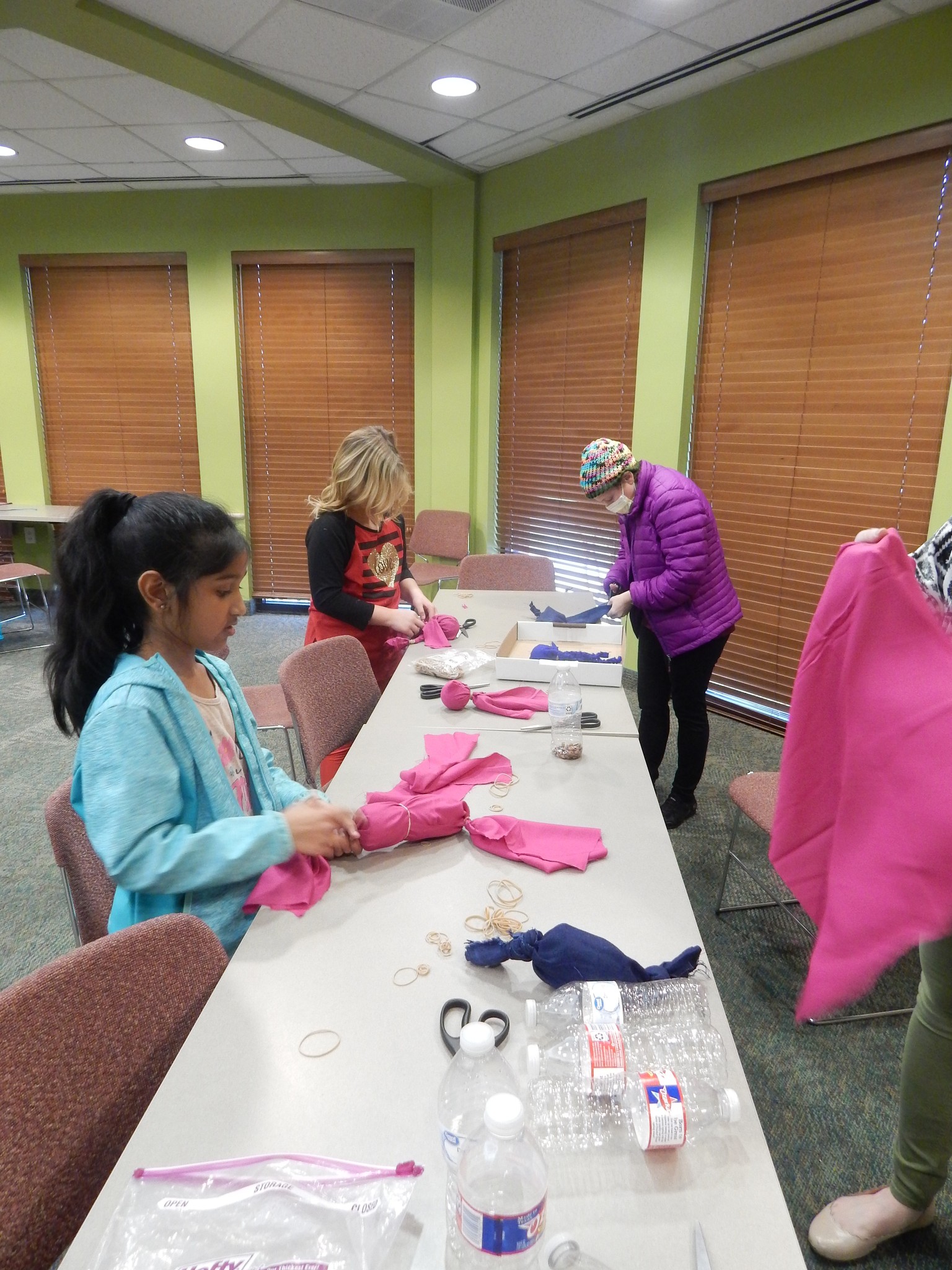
[495,621,626,688]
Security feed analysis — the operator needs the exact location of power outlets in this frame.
[24,527,36,544]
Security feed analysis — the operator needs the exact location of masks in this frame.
[605,481,636,514]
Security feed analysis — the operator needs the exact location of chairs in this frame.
[409,510,471,591]
[204,645,297,783]
[277,634,381,792]
[0,550,56,655]
[0,913,228,1270]
[714,771,913,1026]
[459,554,556,591]
[45,776,116,949]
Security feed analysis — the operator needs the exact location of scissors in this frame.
[456,619,476,638]
[420,683,491,700]
[439,997,510,1055]
[609,583,622,601]
[521,711,601,733]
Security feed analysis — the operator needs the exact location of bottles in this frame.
[456,1091,549,1270]
[436,1021,524,1222]
[547,666,583,760]
[526,977,710,1037]
[524,1023,725,1098]
[519,1065,741,1155]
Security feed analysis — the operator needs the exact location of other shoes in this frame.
[808,1185,935,1260]
[660,793,698,829]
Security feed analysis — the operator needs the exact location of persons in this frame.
[305,426,436,787]
[579,437,743,830]
[42,487,369,964]
[806,518,952,1262]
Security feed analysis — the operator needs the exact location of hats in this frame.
[580,437,639,499]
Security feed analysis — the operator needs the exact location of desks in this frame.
[366,589,641,738]
[0,504,83,522]
[56,724,807,1270]
